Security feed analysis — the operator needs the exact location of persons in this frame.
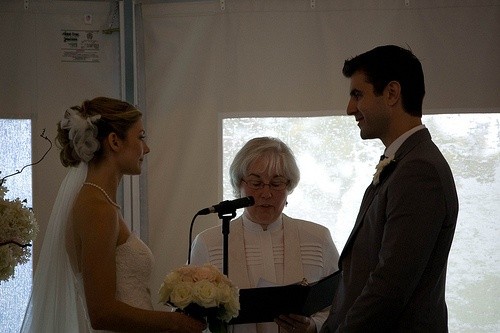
[171,137,343,333]
[319,44,459,333]
[57,97,207,333]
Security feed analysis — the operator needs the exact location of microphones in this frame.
[197,195,255,215]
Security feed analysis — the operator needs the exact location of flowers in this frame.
[159,264,241,326]
[0,170,41,286]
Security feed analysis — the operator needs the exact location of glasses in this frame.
[241,178,290,191]
